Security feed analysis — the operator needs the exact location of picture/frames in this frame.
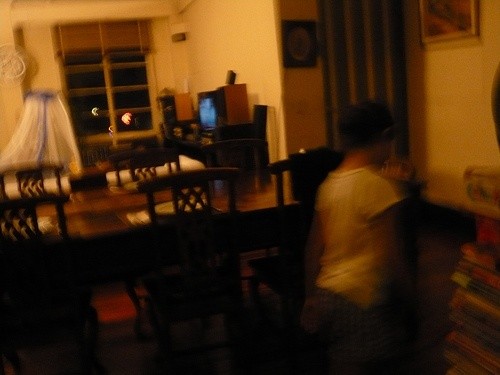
[418,0,480,48]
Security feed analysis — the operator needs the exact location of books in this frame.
[442,164,500,375]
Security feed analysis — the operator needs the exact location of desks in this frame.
[0,168,302,240]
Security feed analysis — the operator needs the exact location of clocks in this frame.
[282,20,317,69]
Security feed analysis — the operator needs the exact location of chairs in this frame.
[0,139,344,375]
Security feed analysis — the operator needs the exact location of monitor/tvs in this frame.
[196,91,226,130]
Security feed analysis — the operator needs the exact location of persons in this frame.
[297,96,412,375]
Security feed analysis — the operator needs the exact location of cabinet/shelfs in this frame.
[162,125,266,172]
